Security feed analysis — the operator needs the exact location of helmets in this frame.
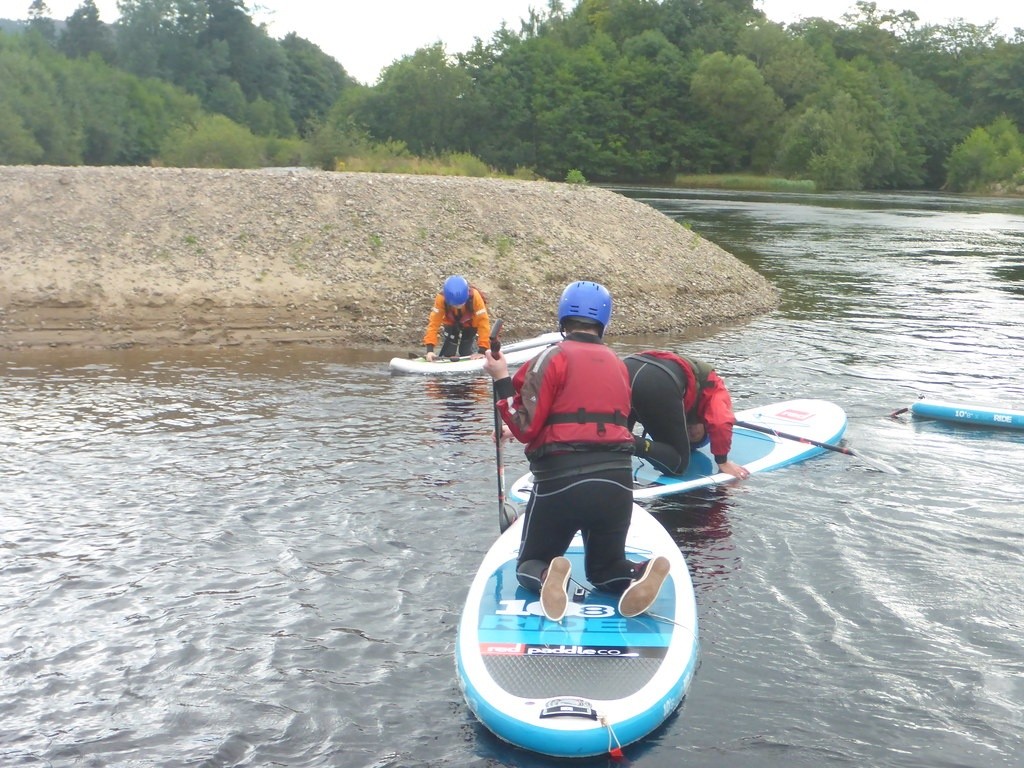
[690,435,710,451]
[558,281,611,339]
[443,274,469,307]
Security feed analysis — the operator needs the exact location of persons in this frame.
[423,275,491,362]
[485,279,671,621]
[621,349,749,479]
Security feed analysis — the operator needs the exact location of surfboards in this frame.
[389,331,568,375]
[912,401,1024,431]
[508,398,847,505]
[453,494,701,759]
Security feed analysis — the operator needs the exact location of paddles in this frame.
[733,420,902,478]
[488,319,520,535]
[451,338,562,363]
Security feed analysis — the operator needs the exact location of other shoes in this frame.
[618,556,670,617]
[540,556,572,622]
